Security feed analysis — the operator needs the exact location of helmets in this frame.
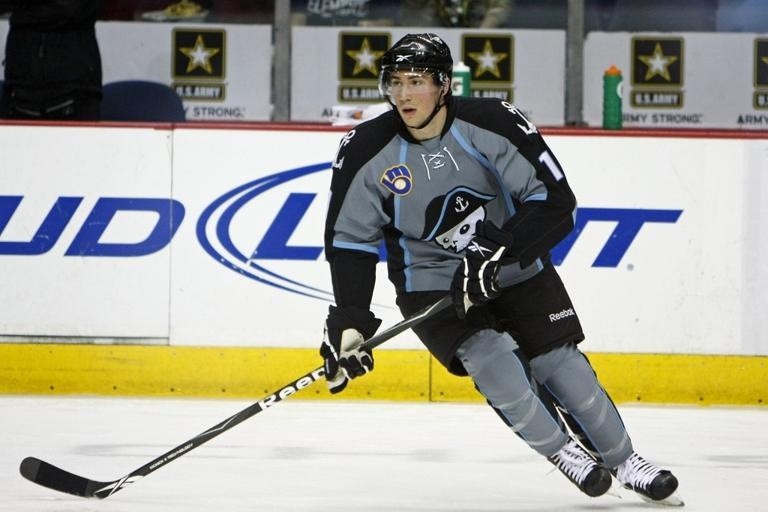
[377,33,454,105]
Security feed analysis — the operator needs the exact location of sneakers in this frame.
[610,450,679,502]
[546,433,612,496]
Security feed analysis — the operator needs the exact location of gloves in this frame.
[320,304,383,395]
[453,248,509,319]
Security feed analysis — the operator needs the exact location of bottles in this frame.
[450,60,472,98]
[602,64,624,130]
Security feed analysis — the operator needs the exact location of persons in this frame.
[2,2,102,121]
[317,29,679,499]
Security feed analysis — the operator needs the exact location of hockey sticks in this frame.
[20,293,454,501]
